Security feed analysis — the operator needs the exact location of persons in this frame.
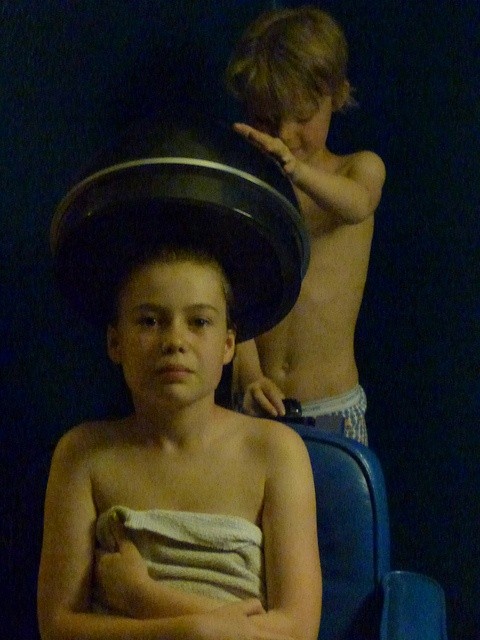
[223,6,389,448]
[36,200,323,640]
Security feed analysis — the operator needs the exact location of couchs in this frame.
[0,420,449,640]
